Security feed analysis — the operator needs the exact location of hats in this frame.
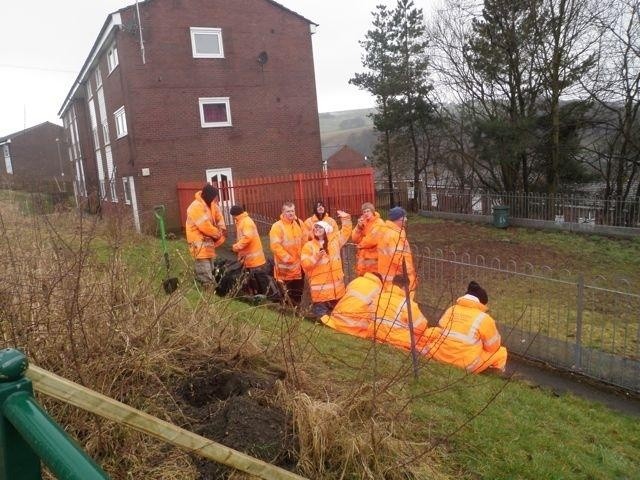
[465,281,488,305]
[388,206,405,220]
[230,206,243,215]
[313,221,333,235]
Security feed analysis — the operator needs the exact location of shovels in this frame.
[153,205,178,295]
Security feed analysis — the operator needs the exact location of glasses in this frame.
[316,226,323,229]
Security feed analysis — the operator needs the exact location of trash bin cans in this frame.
[492,206,510,229]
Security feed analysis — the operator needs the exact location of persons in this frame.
[302,201,340,242]
[364,273,429,352]
[425,280,507,376]
[185,183,227,290]
[376,205,418,302]
[229,205,271,296]
[352,201,385,278]
[268,201,306,307]
[319,271,385,340]
[300,208,352,324]
